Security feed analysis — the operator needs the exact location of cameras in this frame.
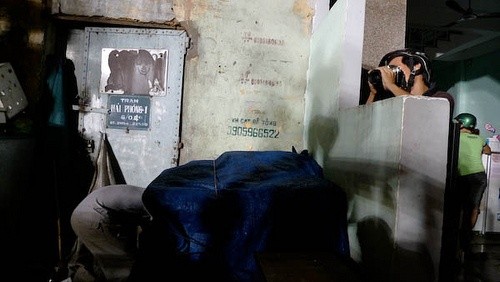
[368,65,406,94]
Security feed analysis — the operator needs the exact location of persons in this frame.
[442,113,491,259]
[365,47,454,121]
[71,185,149,282]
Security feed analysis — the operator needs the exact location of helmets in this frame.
[378,49,432,83]
[455,113,477,129]
